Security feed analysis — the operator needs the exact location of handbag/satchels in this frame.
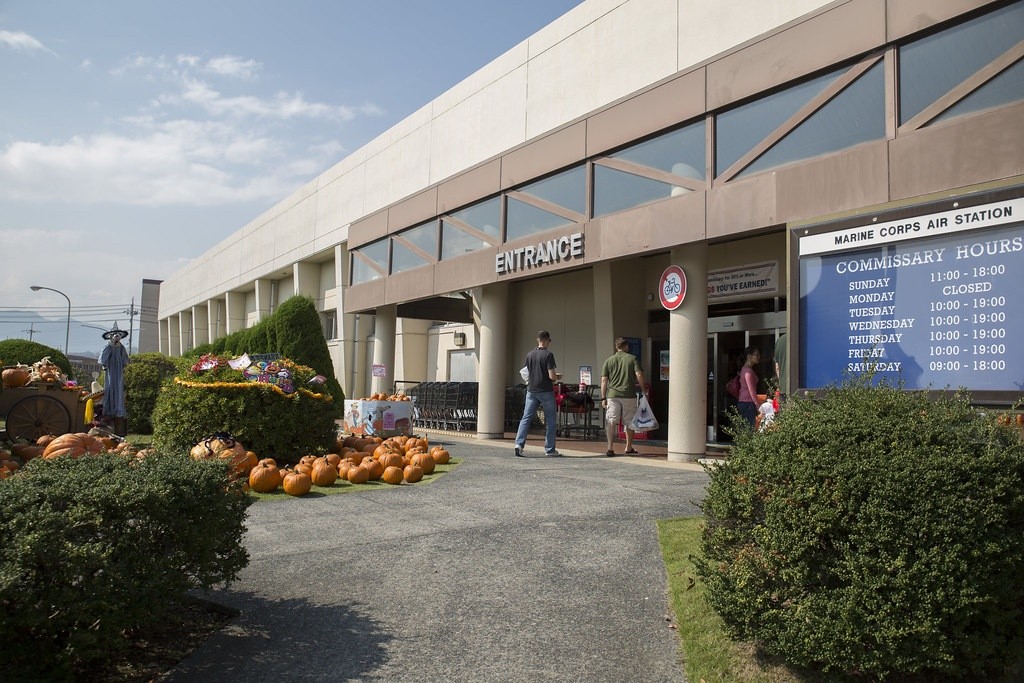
[629,394,659,431]
[725,375,740,399]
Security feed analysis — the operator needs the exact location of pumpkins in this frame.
[0,364,450,500]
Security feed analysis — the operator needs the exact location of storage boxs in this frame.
[344,399,414,440]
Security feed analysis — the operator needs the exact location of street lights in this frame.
[30,285,71,357]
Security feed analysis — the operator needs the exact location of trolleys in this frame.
[403,379,607,441]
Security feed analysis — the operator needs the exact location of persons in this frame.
[513,329,562,457]
[599,336,650,458]
[772,327,788,415]
[755,385,778,438]
[97,319,133,420]
[735,346,762,444]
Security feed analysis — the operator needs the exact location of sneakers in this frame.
[546,450,562,457]
[514,445,526,456]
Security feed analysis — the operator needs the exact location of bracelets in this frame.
[601,396,606,401]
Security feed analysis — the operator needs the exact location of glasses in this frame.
[750,353,761,357]
[549,339,551,342]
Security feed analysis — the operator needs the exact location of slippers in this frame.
[624,449,637,455]
[605,450,613,457]
